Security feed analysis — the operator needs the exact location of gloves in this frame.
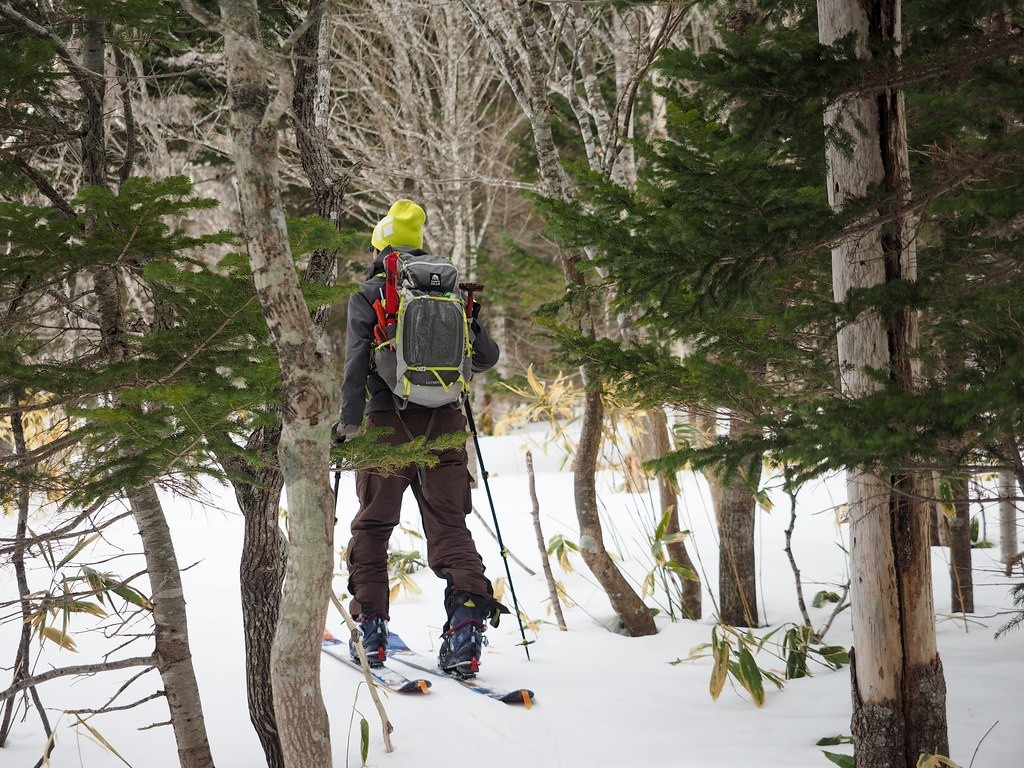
[330,420,360,447]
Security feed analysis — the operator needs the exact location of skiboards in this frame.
[321,626,535,708]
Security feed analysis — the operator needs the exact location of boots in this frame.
[438,586,511,681]
[348,612,390,668]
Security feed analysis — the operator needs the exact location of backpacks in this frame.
[368,250,475,410]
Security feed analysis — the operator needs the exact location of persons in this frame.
[337,199,500,681]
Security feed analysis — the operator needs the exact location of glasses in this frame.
[369,246,374,253]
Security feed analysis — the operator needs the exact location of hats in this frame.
[370,198,427,252]
